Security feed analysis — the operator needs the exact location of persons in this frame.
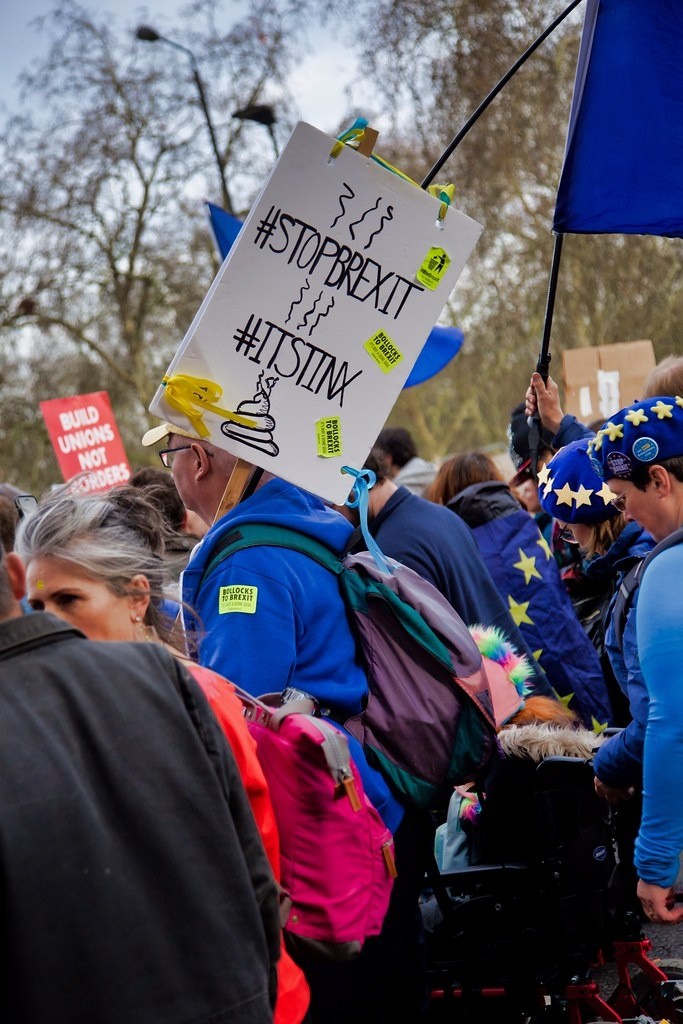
[0,356,682,1024]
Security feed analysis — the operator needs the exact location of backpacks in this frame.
[234,689,398,956]
[203,520,484,805]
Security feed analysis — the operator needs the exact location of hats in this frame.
[587,395,682,476]
[142,420,200,448]
[538,438,621,524]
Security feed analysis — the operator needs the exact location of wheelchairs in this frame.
[408,727,683,1024]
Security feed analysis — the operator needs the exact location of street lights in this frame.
[137,24,235,217]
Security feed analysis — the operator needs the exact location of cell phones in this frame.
[14,495,40,519]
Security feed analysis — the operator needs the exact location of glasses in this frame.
[159,443,211,467]
[613,481,644,515]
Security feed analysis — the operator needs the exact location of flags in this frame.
[552,1,683,237]
[201,199,464,389]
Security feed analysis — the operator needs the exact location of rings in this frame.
[648,911,656,917]
